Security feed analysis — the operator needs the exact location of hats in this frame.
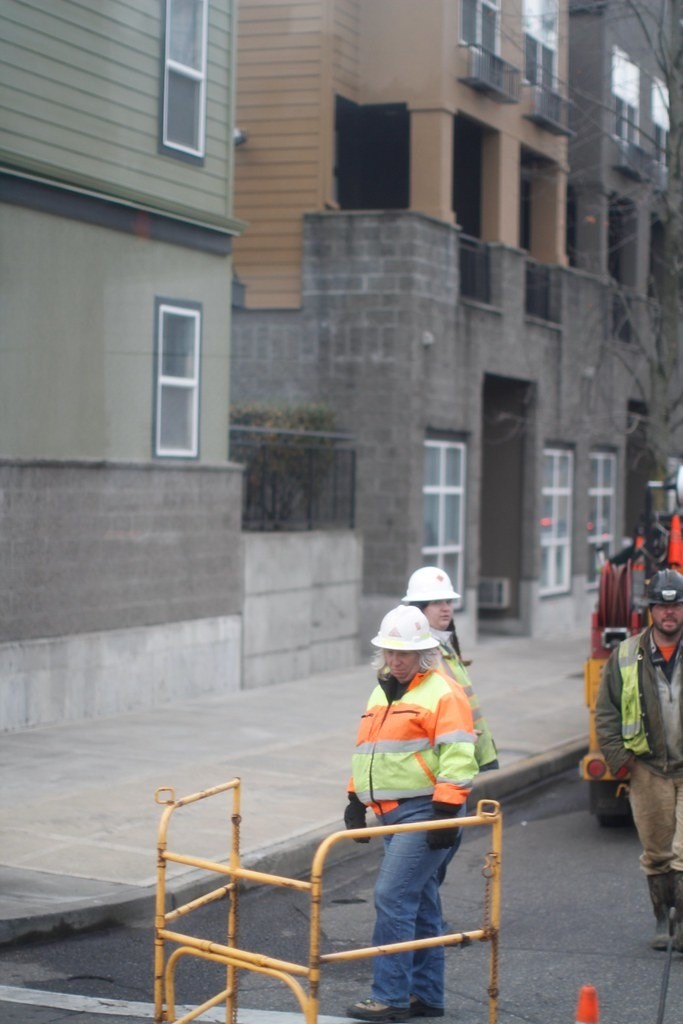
[642,568,683,605]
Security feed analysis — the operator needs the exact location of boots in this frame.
[647,870,683,953]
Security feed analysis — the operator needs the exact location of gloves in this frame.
[344,791,370,843]
[426,803,460,851]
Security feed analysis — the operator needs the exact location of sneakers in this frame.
[393,994,445,1022]
[348,996,410,1023]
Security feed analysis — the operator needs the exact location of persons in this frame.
[595,568,683,954]
[345,565,500,1018]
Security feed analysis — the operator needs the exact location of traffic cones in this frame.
[575,984,600,1024]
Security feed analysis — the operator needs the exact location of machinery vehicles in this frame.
[577,467,682,830]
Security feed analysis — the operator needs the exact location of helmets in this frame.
[401,565,460,601]
[369,605,439,650]
